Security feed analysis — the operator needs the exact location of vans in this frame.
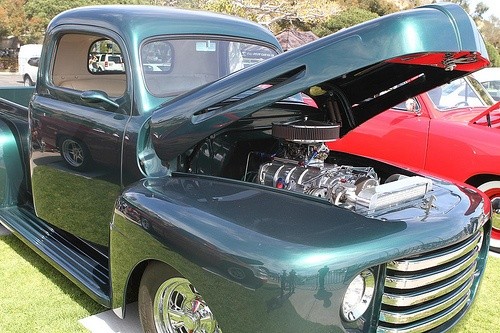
[19,43,42,87]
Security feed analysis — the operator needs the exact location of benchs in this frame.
[60,76,212,98]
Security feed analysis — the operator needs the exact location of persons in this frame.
[280,269,287,293]
[318,264,329,288]
[288,268,297,293]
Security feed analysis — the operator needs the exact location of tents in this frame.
[239,27,319,65]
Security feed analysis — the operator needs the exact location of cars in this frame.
[93,53,125,72]
[0,3,490,332]
[324,75,500,254]
